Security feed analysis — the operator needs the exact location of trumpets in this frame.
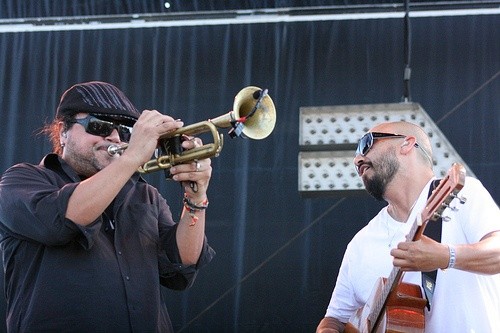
[107,87,277,174]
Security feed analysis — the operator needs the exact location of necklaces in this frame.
[391,200,417,222]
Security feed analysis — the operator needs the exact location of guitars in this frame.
[340,162,467,333]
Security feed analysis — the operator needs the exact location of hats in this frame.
[57,81,140,121]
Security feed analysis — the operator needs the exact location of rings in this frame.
[194,160,201,173]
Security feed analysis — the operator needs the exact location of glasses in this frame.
[69,115,133,143]
[354,132,419,156]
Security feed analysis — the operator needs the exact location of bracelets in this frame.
[447,244,456,271]
[182,194,209,227]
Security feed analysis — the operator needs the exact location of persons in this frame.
[316,122,500,333]
[0,80,212,333]
